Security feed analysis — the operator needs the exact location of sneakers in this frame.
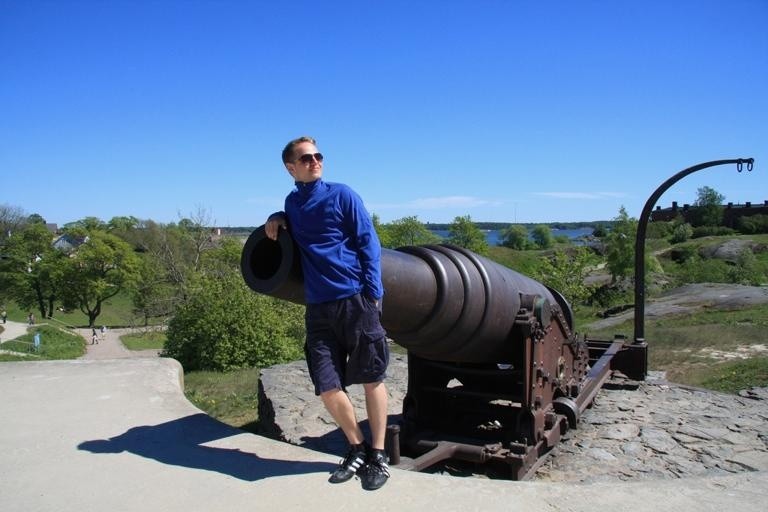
[327,443,371,483]
[364,448,390,490]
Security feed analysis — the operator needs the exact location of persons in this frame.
[101,327,105,340]
[1,310,6,323]
[265,136,390,490]
[29,313,33,325]
[57,306,66,311]
[92,329,98,344]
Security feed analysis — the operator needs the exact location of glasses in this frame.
[294,153,324,164]
